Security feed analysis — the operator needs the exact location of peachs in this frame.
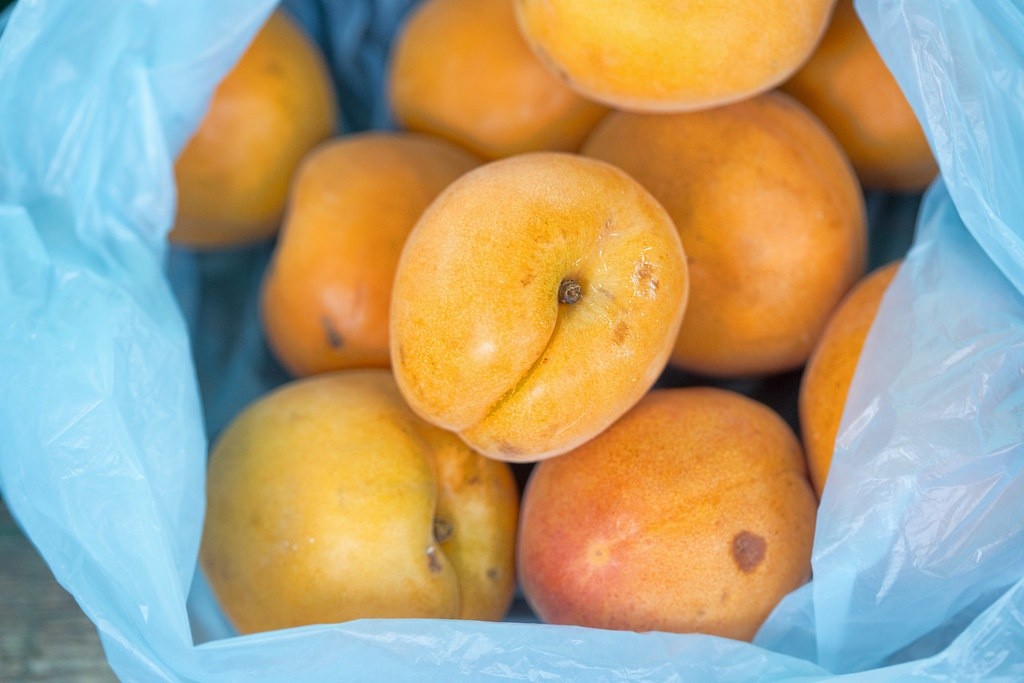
[162,0,1024,646]
